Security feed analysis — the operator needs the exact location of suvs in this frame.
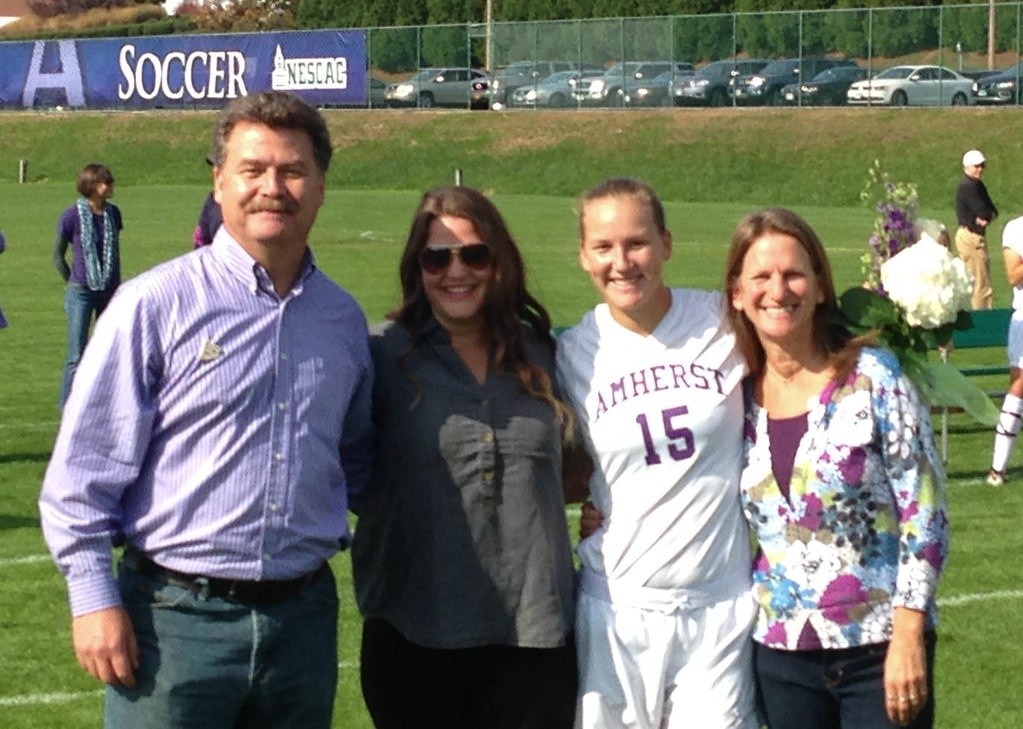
[725,56,867,107]
[667,57,784,107]
[381,65,496,109]
[468,58,695,109]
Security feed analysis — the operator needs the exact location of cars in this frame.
[970,61,1023,107]
[314,75,389,110]
[779,66,886,108]
[846,64,978,107]
[615,69,697,107]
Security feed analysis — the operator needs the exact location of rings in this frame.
[887,695,918,702]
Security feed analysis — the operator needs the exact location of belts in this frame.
[123,550,330,607]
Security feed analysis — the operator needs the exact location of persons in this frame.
[52,164,122,408]
[0,231,9,331]
[951,148,996,312]
[556,177,755,728]
[350,186,592,729]
[986,211,1023,488]
[191,151,225,249]
[38,93,369,729]
[726,211,950,729]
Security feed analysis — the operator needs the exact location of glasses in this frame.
[96,178,114,185]
[418,242,491,275]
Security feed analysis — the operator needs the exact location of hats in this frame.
[963,150,986,166]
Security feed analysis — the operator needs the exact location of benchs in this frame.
[906,309,1013,413]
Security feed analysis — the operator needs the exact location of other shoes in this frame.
[985,466,1004,488]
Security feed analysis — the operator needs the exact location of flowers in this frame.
[877,234,977,332]
[859,155,919,297]
